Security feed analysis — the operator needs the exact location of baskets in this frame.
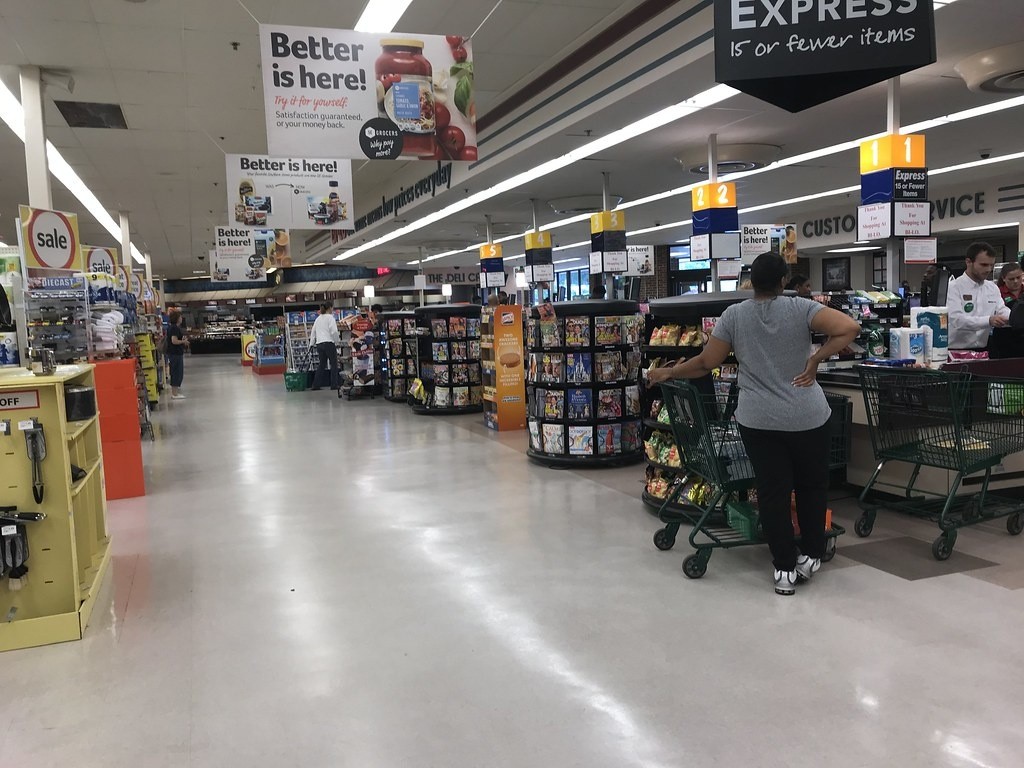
[284,371,309,392]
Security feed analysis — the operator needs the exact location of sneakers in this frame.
[796,553,821,579]
[774,569,797,594]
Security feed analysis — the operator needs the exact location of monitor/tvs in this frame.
[921,268,949,307]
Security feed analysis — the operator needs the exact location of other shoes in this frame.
[171,393,186,399]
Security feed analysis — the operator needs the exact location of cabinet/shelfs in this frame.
[0,204,912,653]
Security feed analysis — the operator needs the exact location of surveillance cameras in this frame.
[454,266,460,270]
[655,222,661,226]
[198,256,205,260]
[977,149,992,159]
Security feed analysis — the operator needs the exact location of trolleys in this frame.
[651,381,854,579]
[850,362,1024,562]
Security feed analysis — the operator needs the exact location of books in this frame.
[432,317,482,404]
[388,319,418,394]
[526,303,641,453]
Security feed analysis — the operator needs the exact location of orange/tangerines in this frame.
[780,230,797,263]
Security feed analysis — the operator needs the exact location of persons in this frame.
[438,345,443,351]
[165,311,190,399]
[612,324,620,337]
[436,323,442,335]
[308,302,340,390]
[590,286,606,298]
[997,252,1024,340]
[459,319,465,323]
[470,342,475,351]
[531,355,535,380]
[367,304,382,328]
[649,252,860,595]
[944,242,1010,350]
[573,325,582,337]
[498,291,508,304]
[567,321,574,332]
[787,276,811,295]
[546,393,551,403]
[473,296,482,304]
[542,355,552,378]
[551,396,558,409]
[923,262,949,287]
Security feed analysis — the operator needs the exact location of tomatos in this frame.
[379,73,402,90]
[417,101,477,161]
[446,35,467,62]
